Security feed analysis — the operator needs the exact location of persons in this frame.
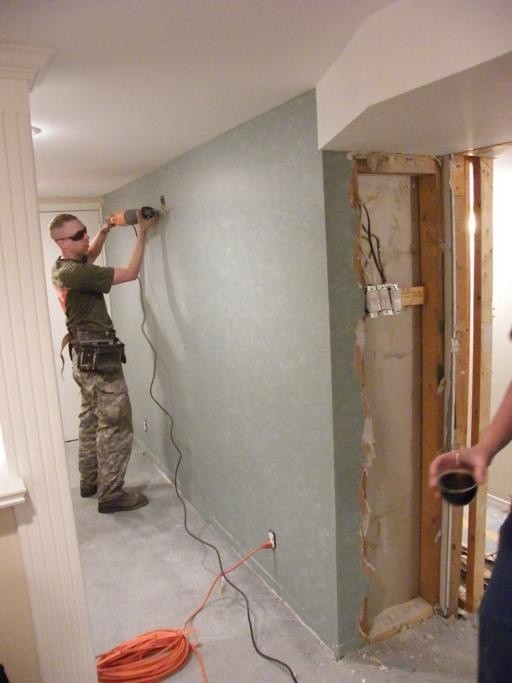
[49,209,160,513]
[429,381,512,683]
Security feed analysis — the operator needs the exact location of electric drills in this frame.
[103,206,162,227]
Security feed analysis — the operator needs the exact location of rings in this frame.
[455,452,460,467]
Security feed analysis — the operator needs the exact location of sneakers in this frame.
[98,492,149,514]
[81,486,97,498]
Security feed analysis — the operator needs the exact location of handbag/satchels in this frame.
[71,339,126,373]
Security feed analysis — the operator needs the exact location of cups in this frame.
[434,446,477,508]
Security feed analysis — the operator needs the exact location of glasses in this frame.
[56,227,87,241]
